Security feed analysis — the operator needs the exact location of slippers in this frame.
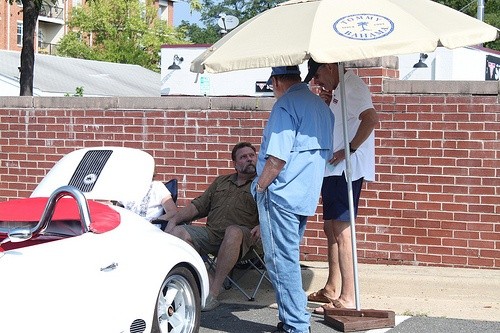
[314,298,345,314]
[307,289,334,302]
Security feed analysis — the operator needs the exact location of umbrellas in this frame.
[190,0,500,310]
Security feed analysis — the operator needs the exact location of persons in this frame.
[165,143,262,311]
[115,181,177,230]
[250,65,335,333]
[304,58,376,315]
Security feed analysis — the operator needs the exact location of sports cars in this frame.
[0,145,209,333]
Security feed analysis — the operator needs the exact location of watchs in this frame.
[349,143,356,153]
[256,184,264,191]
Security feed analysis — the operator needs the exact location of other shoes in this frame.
[278,321,287,333]
[203,296,221,311]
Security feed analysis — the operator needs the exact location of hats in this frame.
[304,58,320,84]
[266,64,300,85]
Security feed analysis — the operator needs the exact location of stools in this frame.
[202,246,273,301]
